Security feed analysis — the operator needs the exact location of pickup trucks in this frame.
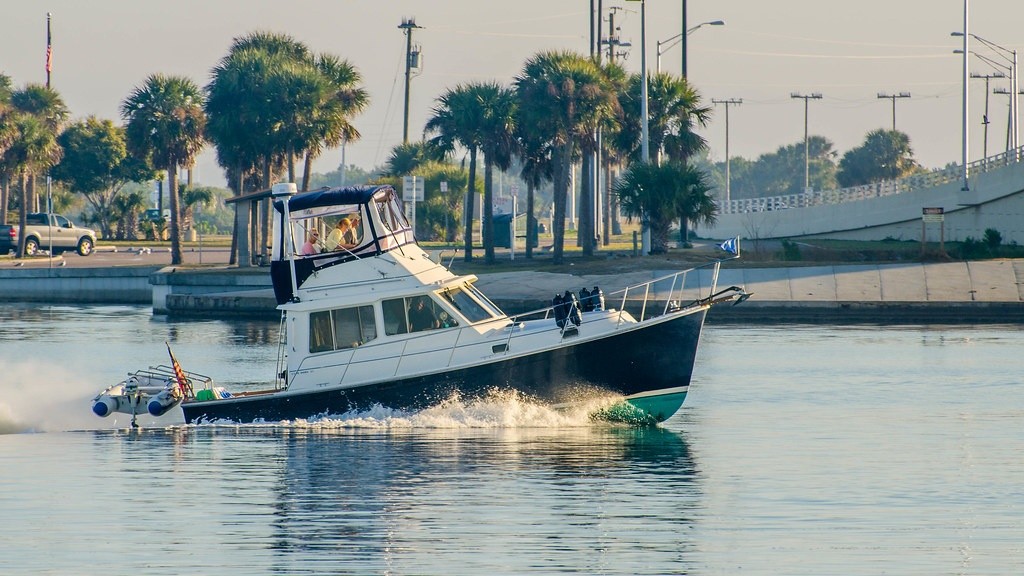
[0,212,97,256]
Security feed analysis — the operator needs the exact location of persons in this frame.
[408,297,440,333]
[302,213,361,255]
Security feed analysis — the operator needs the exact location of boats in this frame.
[92,182,756,431]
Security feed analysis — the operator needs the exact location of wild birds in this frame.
[981,114,990,124]
[58,259,66,267]
[14,261,24,267]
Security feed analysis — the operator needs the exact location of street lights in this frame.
[952,48,1014,150]
[656,19,725,75]
[950,32,1020,163]
